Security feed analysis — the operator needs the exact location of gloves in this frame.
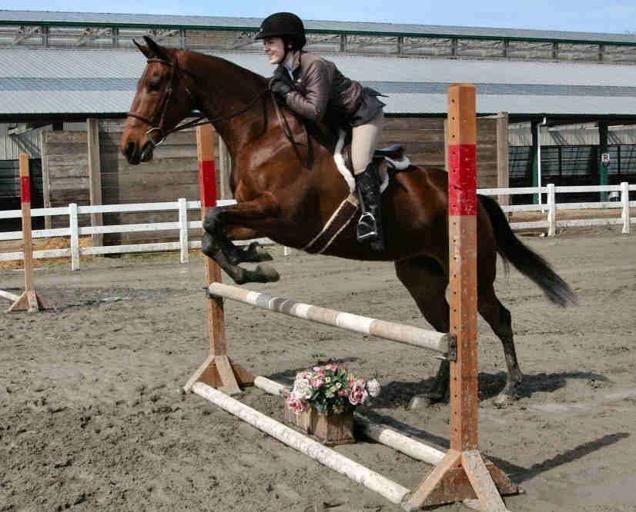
[266,79,292,96]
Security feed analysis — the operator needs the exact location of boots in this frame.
[353,163,385,243]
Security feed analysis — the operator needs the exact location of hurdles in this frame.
[183,84,518,512]
[0,153,50,314]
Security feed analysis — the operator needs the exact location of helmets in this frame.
[253,12,306,41]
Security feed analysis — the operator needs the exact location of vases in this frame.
[285,414,359,445]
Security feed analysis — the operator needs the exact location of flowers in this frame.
[285,360,382,414]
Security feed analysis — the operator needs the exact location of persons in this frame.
[254,12,387,245]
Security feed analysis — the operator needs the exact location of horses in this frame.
[117,34,583,411]
